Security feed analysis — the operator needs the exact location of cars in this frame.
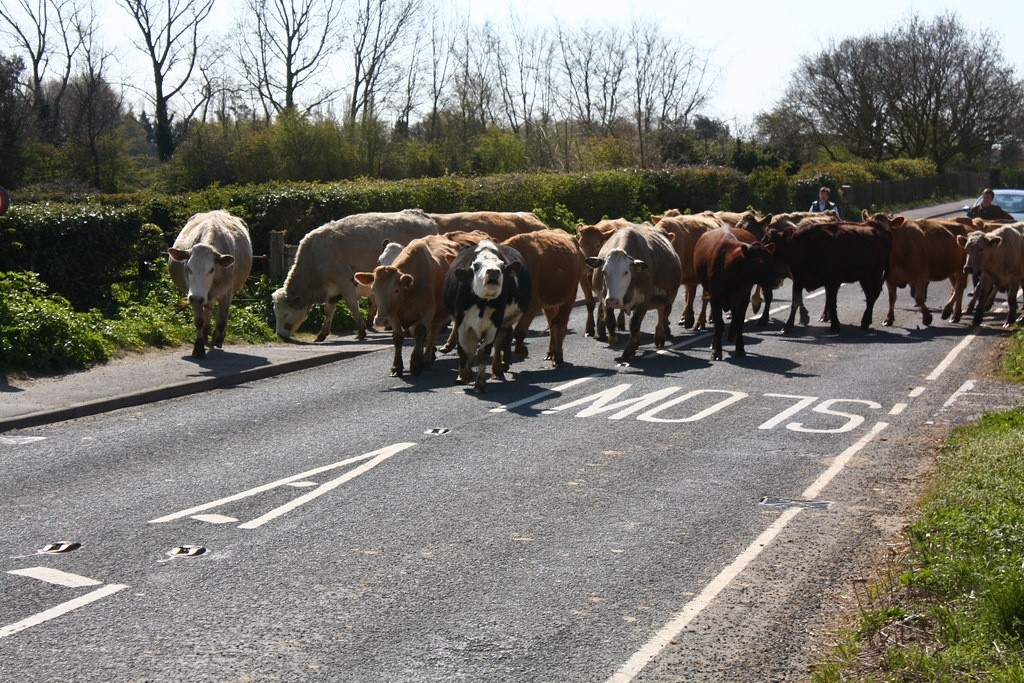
[961,189,1024,221]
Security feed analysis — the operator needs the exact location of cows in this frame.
[166,207,255,360]
[270,206,1024,395]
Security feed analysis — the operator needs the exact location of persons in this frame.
[967,188,1014,295]
[809,188,838,218]
